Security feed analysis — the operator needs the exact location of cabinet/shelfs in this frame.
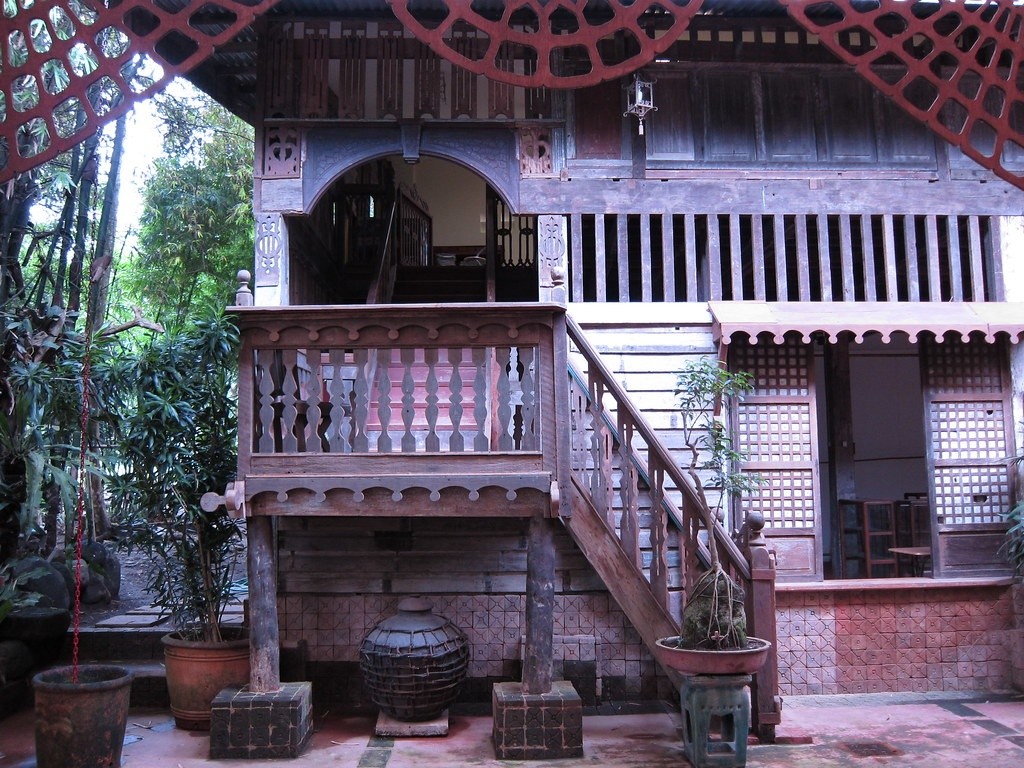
[895,499,932,574]
[838,497,899,579]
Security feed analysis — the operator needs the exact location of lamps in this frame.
[621,68,661,137]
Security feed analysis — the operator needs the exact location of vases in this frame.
[29,664,131,767]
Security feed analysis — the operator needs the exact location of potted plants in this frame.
[654,353,774,676]
[12,301,253,734]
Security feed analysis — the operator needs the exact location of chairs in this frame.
[888,546,932,577]
